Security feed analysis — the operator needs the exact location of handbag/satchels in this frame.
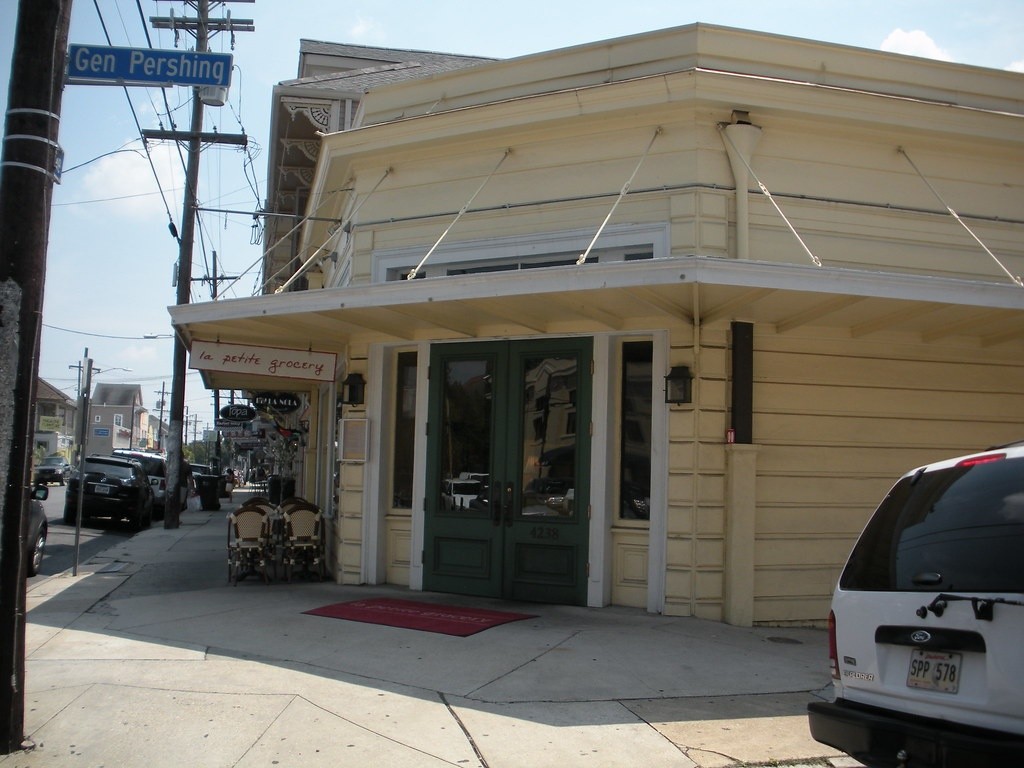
[186,489,203,511]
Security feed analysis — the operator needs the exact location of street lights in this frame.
[76,367,134,450]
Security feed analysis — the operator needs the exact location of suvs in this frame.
[63,452,159,534]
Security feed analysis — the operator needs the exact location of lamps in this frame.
[340,373,366,406]
[663,365,693,406]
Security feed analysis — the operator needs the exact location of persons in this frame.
[164,451,192,525]
[259,466,265,486]
[250,468,258,484]
[225,468,234,503]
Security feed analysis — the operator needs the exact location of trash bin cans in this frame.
[218,475,230,499]
[267,474,280,506]
[195,475,222,511]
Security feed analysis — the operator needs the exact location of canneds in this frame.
[727,429,735,444]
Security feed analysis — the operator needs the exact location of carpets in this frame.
[300,599,541,637]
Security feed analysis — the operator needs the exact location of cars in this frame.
[807,440,1023,768]
[32,455,74,487]
[441,479,488,511]
[28,485,50,578]
[110,449,166,521]
[469,489,560,518]
[181,460,211,513]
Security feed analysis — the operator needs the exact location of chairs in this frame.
[226,495,326,587]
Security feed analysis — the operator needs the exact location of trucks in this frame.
[461,473,490,490]
